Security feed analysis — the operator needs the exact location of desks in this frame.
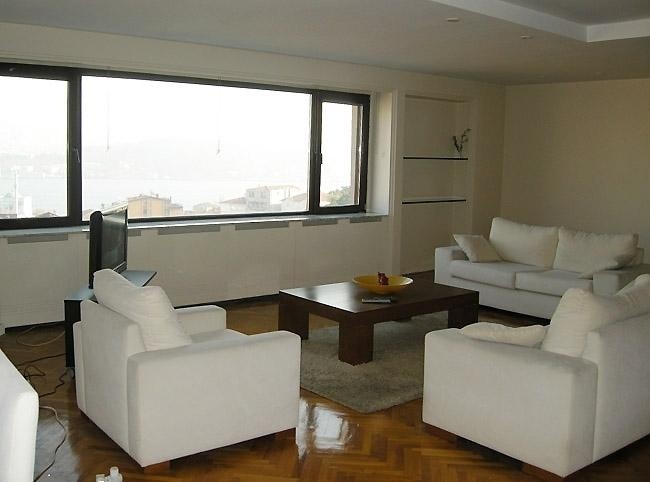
[63,269,160,369]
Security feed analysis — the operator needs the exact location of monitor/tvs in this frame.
[88,204,128,290]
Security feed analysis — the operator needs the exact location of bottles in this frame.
[96,466,122,482]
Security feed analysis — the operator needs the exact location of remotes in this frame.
[362,297,391,304]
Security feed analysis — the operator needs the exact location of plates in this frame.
[353,276,413,294]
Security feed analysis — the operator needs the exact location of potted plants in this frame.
[447,126,472,158]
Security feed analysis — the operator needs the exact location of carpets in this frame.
[267,310,526,414]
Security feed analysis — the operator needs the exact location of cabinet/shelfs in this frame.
[402,155,471,205]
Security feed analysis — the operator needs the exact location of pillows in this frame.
[460,320,546,350]
[452,231,501,268]
[552,227,641,278]
[89,264,192,355]
[487,217,558,270]
[537,275,649,359]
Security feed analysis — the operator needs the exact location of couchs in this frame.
[418,313,650,482]
[0,342,39,481]
[69,297,301,469]
[433,216,646,322]
